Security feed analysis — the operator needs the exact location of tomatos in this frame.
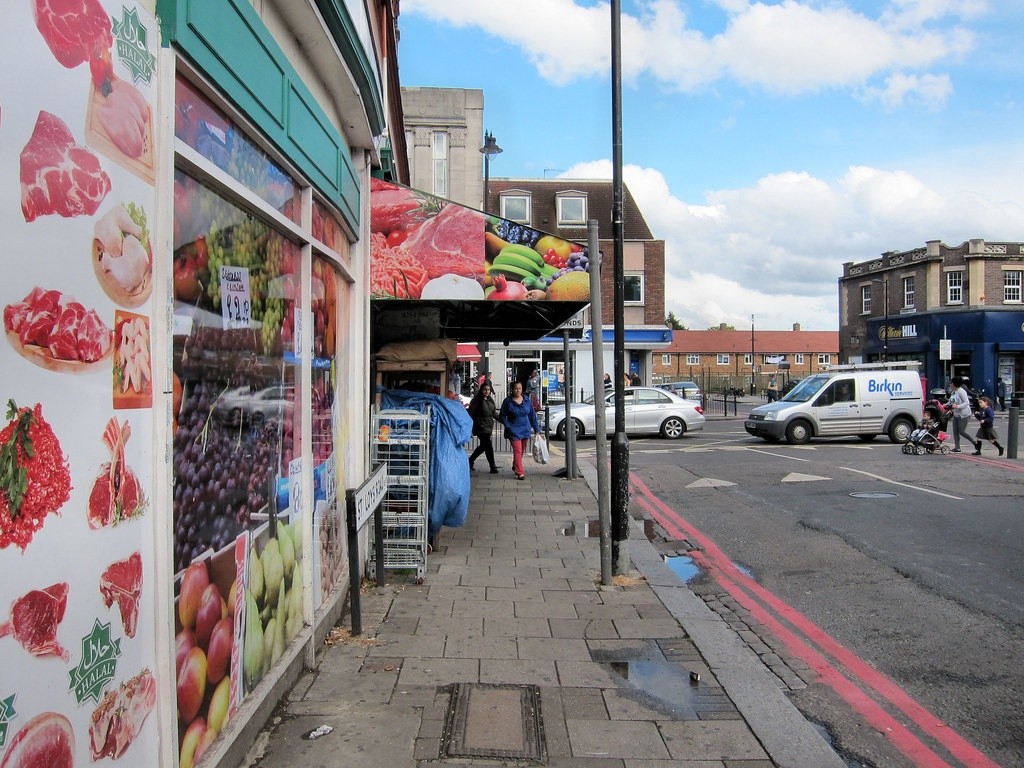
[386,230,406,247]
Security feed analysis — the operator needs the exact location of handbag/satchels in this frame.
[533,433,549,464]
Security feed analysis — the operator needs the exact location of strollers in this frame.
[899,399,952,456]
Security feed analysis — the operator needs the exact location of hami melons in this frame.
[544,271,590,301]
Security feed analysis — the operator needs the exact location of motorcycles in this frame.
[929,384,993,416]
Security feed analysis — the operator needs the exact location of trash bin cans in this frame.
[1011,391,1024,415]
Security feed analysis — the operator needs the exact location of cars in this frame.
[218,385,298,428]
[541,387,706,440]
[782,379,817,399]
[652,382,703,401]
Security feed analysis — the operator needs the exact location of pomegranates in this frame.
[486,273,528,300]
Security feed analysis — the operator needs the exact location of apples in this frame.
[175,83,326,358]
[175,561,236,768]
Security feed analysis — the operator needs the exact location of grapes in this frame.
[173,140,293,571]
[542,243,590,286]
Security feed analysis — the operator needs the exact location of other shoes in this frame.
[911,436,919,441]
[490,468,499,474]
[518,474,526,480]
[906,435,911,440]
[469,458,475,470]
[950,449,962,453]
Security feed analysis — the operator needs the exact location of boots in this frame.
[993,441,1004,455]
[972,441,983,455]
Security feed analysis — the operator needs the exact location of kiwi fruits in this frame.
[521,288,546,300]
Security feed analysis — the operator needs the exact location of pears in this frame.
[243,519,304,696]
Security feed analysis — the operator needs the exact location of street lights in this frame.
[476,129,505,384]
[871,278,889,362]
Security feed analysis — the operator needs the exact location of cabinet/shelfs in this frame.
[367,400,432,583]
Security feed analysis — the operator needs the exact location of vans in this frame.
[744,360,926,443]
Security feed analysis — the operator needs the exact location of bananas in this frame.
[488,243,559,284]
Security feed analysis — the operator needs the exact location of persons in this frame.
[767,374,778,402]
[624,373,630,386]
[835,384,850,401]
[942,376,978,453]
[558,370,564,393]
[972,397,1004,456]
[961,376,977,397]
[499,381,541,480]
[604,373,612,402]
[479,371,496,394]
[468,382,501,473]
[630,373,641,386]
[905,411,934,442]
[997,377,1007,411]
[526,370,540,403]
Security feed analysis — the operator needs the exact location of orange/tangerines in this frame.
[535,236,572,259]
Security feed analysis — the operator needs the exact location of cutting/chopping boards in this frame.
[93,238,153,308]
[6,326,115,376]
[84,76,157,186]
[112,310,153,410]
